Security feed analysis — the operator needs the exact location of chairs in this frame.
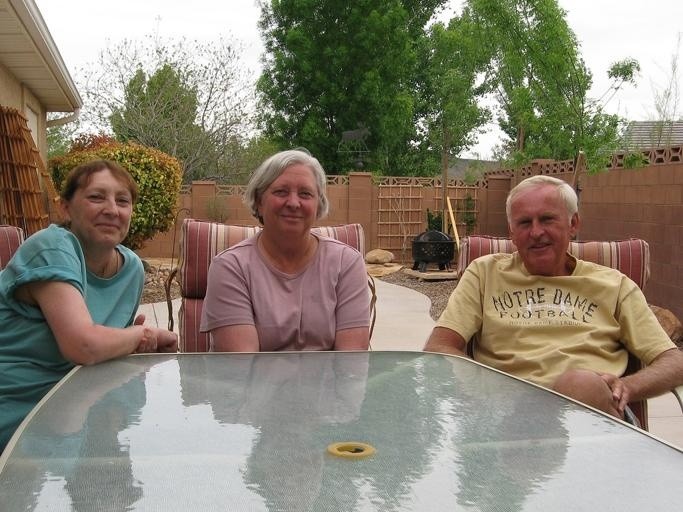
[166,216,376,353]
[459,237,653,435]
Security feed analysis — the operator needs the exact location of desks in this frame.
[0,348,683,512]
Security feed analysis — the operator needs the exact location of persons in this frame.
[199,149,370,355]
[422,174,683,430]
[0,161,178,458]
